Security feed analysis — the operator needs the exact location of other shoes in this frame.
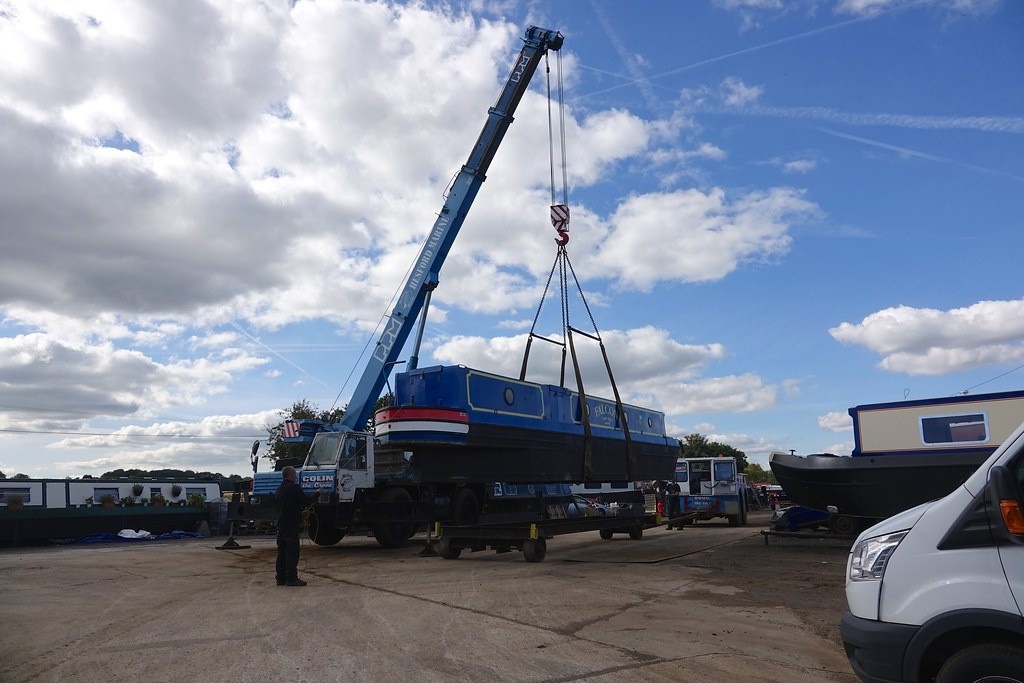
[666,527,673,530]
[286,579,307,586]
[677,528,683,530]
[277,578,286,586]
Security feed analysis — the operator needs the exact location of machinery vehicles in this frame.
[665,455,749,528]
[216,23,572,560]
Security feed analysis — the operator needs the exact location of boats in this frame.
[768,443,1005,524]
[372,363,682,487]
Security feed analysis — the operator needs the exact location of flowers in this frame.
[141,498,149,504]
[6,495,22,505]
[85,496,93,504]
[151,493,164,503]
[100,494,115,502]
[129,495,137,503]
[119,497,127,505]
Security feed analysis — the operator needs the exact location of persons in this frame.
[666,480,683,530]
[276,466,323,587]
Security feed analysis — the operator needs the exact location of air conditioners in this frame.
[84,474,91,480]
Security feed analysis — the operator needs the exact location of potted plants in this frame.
[166,500,169,506]
[178,499,186,507]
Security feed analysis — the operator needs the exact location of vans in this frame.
[838,420,1024,683]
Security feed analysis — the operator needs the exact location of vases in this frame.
[144,502,148,507]
[76,504,81,508]
[87,504,92,508]
[129,503,136,506]
[122,503,126,507]
[154,502,163,507]
[102,501,115,508]
[8,502,20,510]
